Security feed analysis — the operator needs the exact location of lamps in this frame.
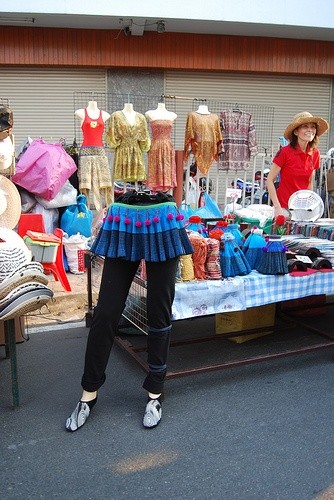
[157,23,165,33]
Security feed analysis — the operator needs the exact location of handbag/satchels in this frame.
[141,229,222,283]
[11,139,93,274]
[325,167,334,191]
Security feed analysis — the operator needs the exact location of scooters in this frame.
[230,169,281,208]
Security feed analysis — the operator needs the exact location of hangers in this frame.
[233,103,239,111]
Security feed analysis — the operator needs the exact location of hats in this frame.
[0,103,54,322]
[284,112,329,142]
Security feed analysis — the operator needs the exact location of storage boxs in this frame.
[216,302,275,343]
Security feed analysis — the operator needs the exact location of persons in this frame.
[63,191,191,433]
[109,102,151,182]
[145,102,178,192]
[265,111,330,219]
[75,100,112,190]
[183,104,225,174]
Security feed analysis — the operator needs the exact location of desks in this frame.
[84,249,334,380]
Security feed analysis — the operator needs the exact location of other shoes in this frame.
[65,396,97,432]
[143,394,165,429]
